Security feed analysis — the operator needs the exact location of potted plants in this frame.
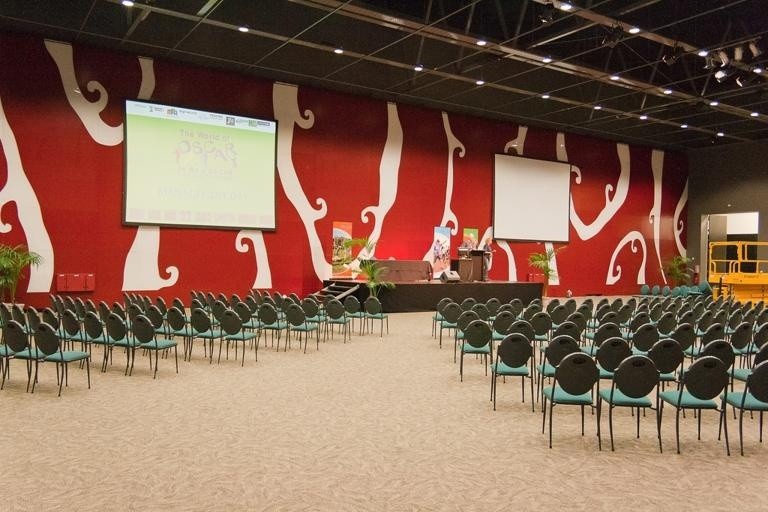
[526,243,561,309]
[0,241,48,314]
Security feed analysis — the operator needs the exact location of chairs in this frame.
[0,287,390,401]
[429,294,768,456]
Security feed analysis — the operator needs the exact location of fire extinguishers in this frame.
[693,272,699,286]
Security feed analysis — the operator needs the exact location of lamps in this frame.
[531,0,768,90]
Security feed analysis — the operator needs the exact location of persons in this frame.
[433,239,450,262]
[483,238,497,282]
[460,233,479,258]
